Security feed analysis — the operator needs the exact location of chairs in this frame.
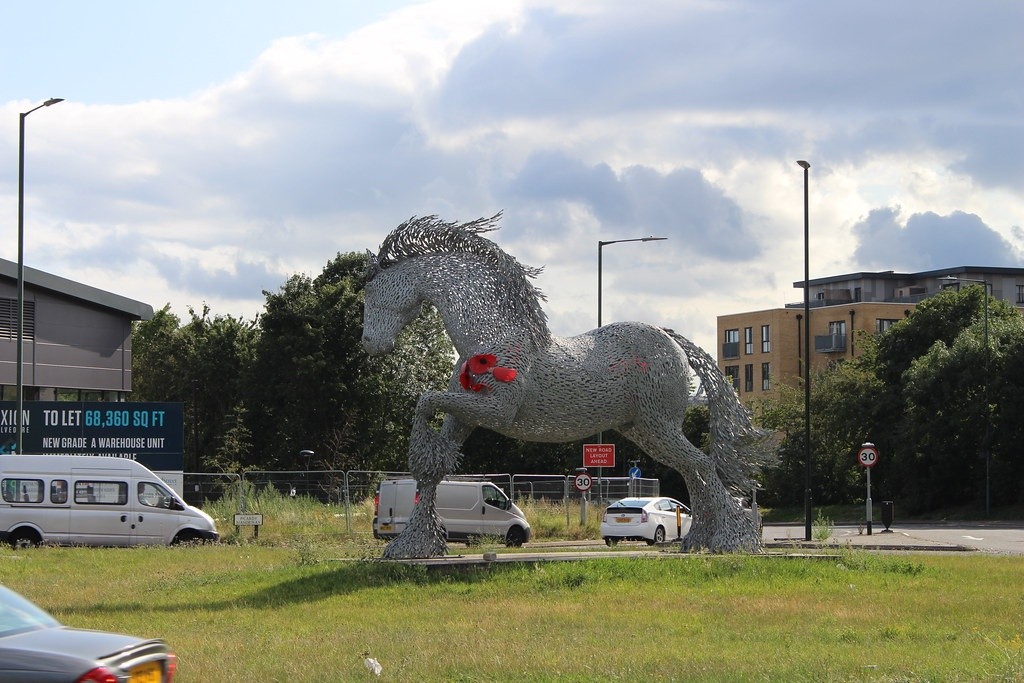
[87,486,97,502]
[23,485,29,501]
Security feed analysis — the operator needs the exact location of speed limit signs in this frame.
[857,446,877,467]
[574,475,592,490]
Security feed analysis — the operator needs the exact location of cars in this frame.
[0,583,178,683]
[600,496,694,546]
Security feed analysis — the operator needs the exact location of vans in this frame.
[0,455,222,551]
[372,480,532,547]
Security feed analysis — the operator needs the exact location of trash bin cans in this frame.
[881,501,895,533]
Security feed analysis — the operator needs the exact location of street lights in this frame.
[12,97,66,455]
[597,236,668,328]
[937,274,991,518]
[796,159,814,542]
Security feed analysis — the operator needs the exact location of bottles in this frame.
[364,656,382,677]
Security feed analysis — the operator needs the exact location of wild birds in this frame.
[364,657,382,677]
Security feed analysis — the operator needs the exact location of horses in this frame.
[361,208,785,560]
